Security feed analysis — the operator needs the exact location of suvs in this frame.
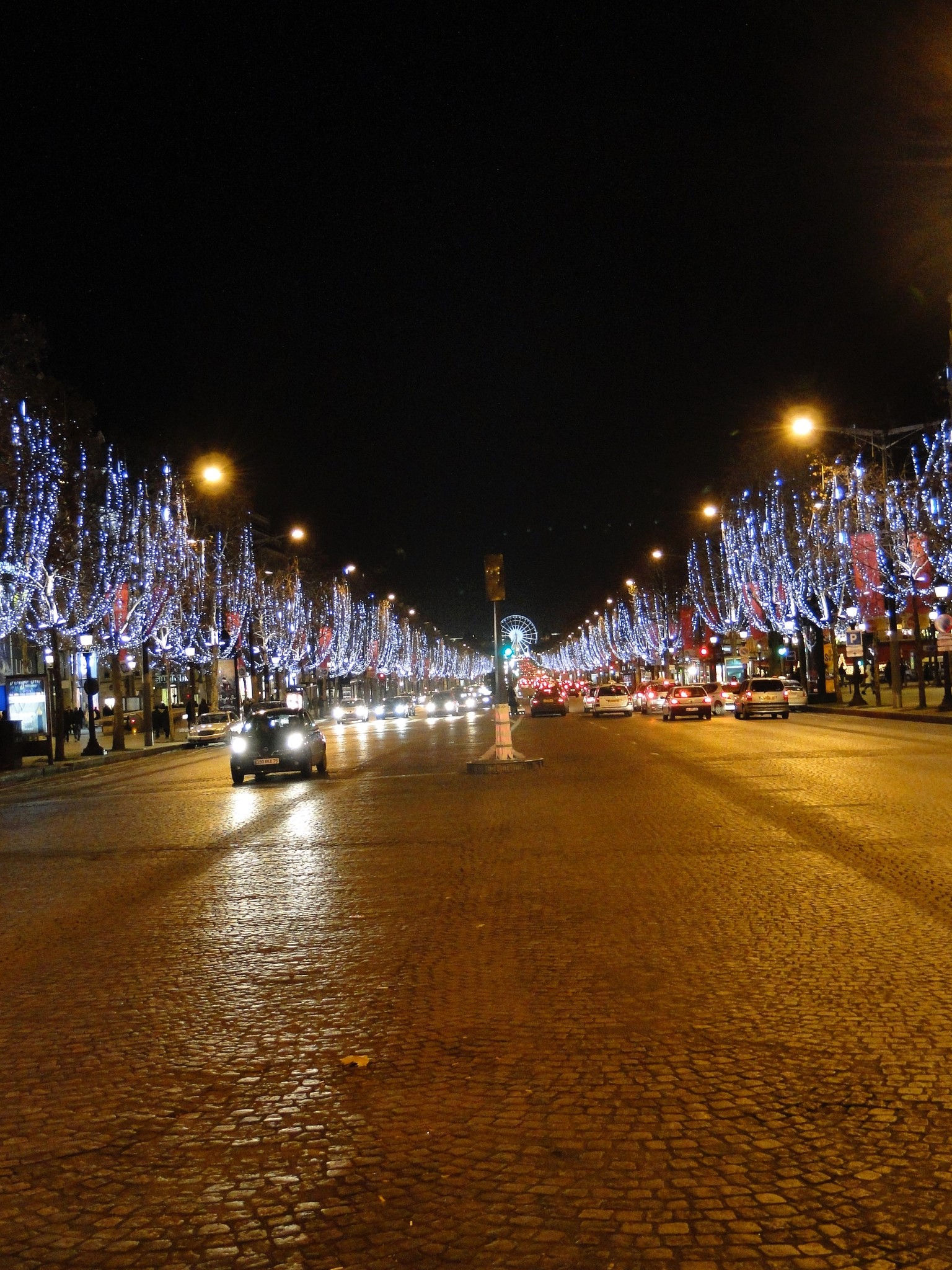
[733,677,790,720]
[245,700,289,723]
[591,683,634,718]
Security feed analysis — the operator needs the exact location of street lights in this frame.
[846,607,867,706]
[933,585,952,712]
[45,655,57,737]
[789,416,952,709]
[185,647,196,728]
[710,637,717,682]
[80,634,107,756]
[740,631,748,680]
[668,647,674,679]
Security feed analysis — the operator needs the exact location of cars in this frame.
[335,697,368,725]
[781,679,807,712]
[702,682,742,716]
[515,676,682,717]
[661,685,712,721]
[187,710,240,748]
[374,683,494,720]
[229,708,326,785]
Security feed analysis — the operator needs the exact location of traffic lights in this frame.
[778,647,788,656]
[502,636,513,656]
[701,649,707,663]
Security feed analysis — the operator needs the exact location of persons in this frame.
[787,667,800,686]
[65,704,115,743]
[507,685,517,716]
[924,665,931,687]
[220,677,236,708]
[858,659,912,695]
[151,696,326,738]
[839,662,847,688]
[732,674,760,681]
